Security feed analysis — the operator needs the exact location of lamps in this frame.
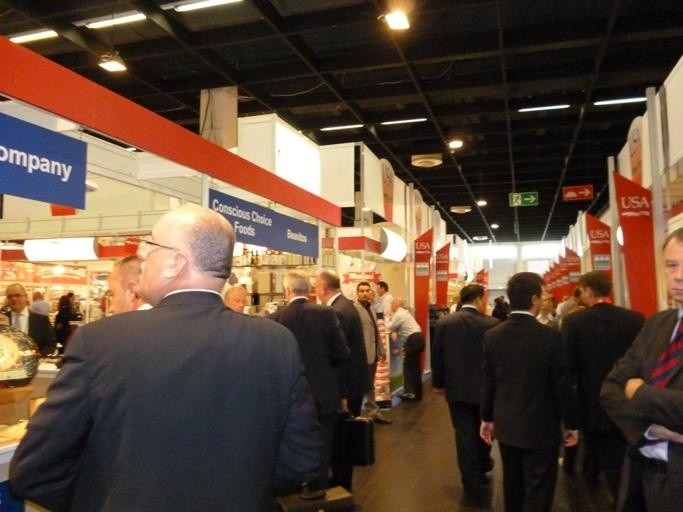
[96,49,128,73]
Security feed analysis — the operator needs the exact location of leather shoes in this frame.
[399,393,416,400]
[369,413,391,424]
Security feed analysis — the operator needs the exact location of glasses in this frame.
[138,236,179,252]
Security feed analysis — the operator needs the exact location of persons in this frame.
[224,286,247,313]
[106,256,154,315]
[314,270,370,491]
[431,284,512,508]
[9,203,330,512]
[377,282,393,315]
[255,270,352,473]
[480,272,579,508]
[369,289,382,321]
[598,228,683,508]
[537,271,646,508]
[354,282,392,424]
[0,283,83,350]
[383,297,425,401]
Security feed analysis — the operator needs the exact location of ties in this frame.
[15,313,22,329]
[651,317,683,387]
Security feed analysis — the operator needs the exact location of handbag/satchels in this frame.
[333,417,374,467]
